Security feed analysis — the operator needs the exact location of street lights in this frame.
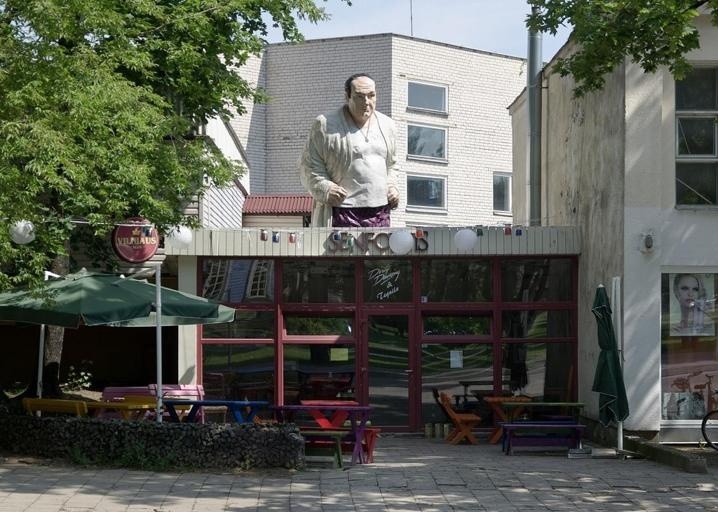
[156,225,194,427]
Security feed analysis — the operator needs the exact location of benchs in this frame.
[24,380,229,430]
[300,422,381,465]
[496,419,587,435]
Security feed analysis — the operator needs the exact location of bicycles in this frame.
[670,369,718,419]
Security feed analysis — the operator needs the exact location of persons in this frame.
[669,273,714,336]
[40,361,71,418]
[297,72,402,229]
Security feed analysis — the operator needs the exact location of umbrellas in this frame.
[0,262,236,422]
[590,275,630,450]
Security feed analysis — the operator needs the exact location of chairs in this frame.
[485,395,529,445]
[440,392,482,445]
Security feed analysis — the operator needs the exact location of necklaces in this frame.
[346,104,373,143]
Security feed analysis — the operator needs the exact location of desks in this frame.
[162,392,372,471]
[500,400,585,460]
